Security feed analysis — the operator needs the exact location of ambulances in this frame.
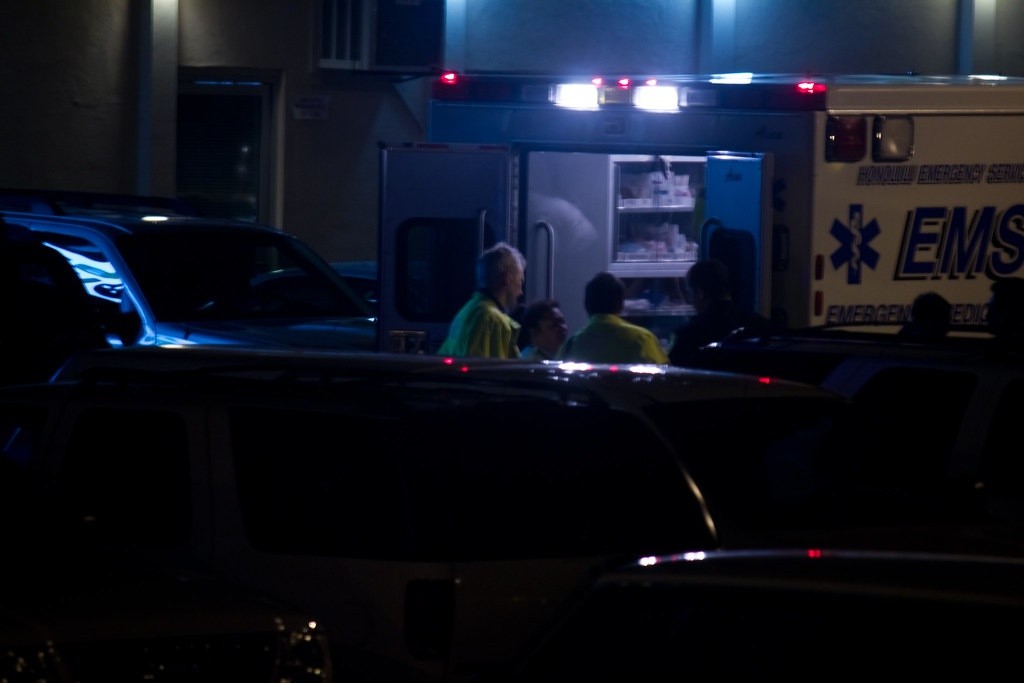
[379,68,1024,353]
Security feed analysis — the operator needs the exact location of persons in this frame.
[667,258,768,375]
[985,277,1024,338]
[555,272,671,365]
[520,298,569,360]
[897,293,953,338]
[438,242,527,360]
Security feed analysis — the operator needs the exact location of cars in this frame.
[0,209,1024,683]
